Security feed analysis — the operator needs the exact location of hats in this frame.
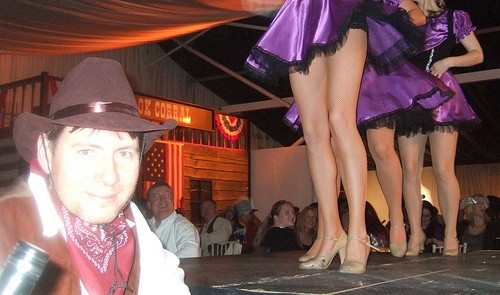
[13,56,178,164]
[235,200,253,213]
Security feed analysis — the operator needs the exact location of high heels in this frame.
[389,222,408,257]
[406,232,426,256]
[298,235,323,262]
[339,234,380,273]
[299,230,348,270]
[442,236,459,256]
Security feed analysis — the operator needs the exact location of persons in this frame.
[132,181,202,259]
[197,200,234,256]
[0,57,192,295]
[241,0,483,273]
[235,201,262,252]
[387,195,495,254]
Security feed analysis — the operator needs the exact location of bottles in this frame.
[0,239,50,295]
